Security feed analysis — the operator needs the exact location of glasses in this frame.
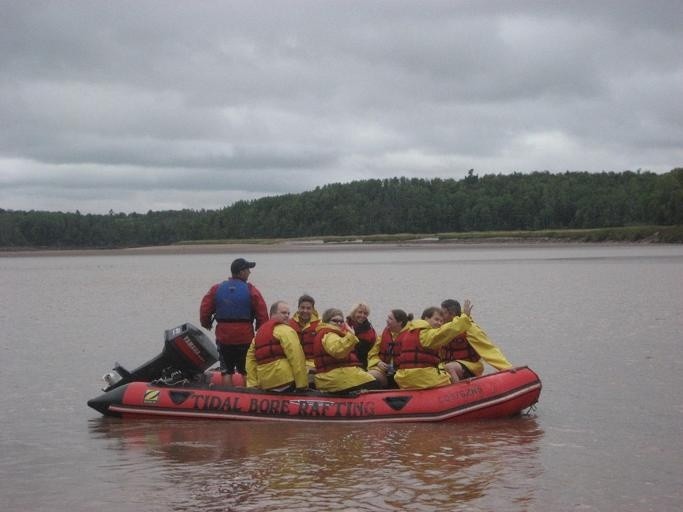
[330,319,344,323]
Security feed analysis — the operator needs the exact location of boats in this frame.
[86,322,543,423]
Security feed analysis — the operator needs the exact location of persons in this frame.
[244,295,514,393]
[200,259,271,388]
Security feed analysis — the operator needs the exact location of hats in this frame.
[231,259,256,272]
